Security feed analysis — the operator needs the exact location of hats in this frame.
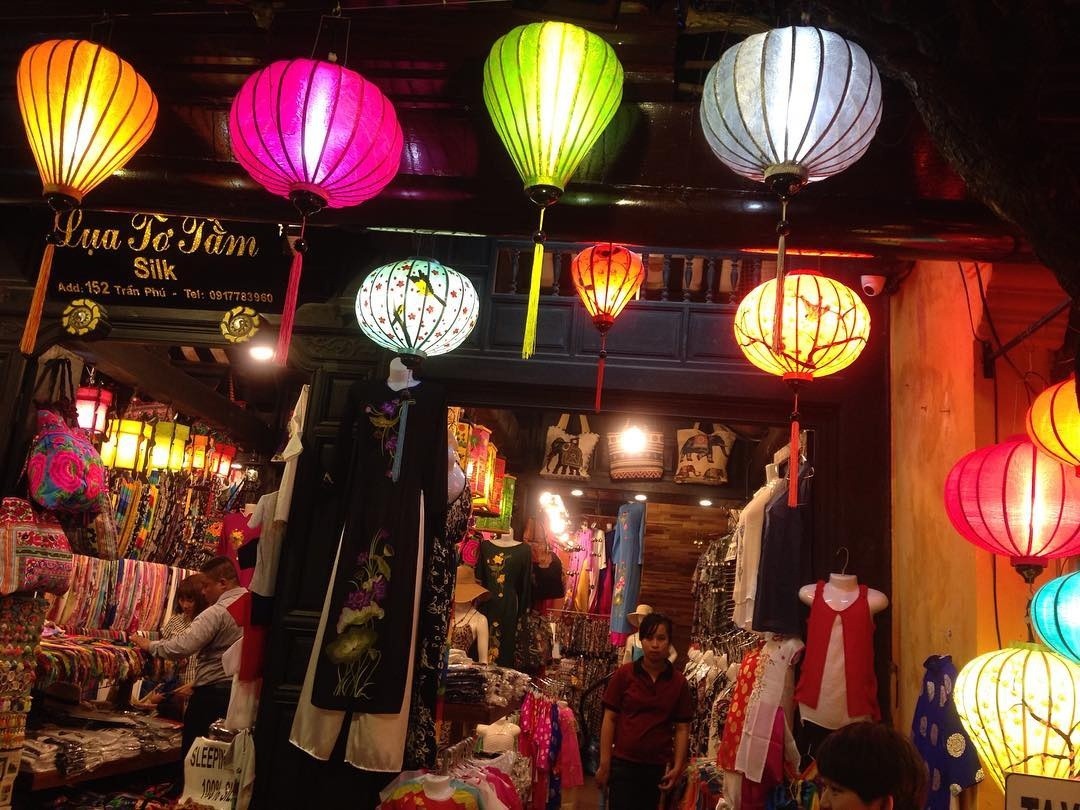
[627,605,652,626]
[453,564,489,604]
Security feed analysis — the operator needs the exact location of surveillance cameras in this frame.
[861,274,886,296]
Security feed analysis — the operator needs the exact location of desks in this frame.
[444,693,526,724]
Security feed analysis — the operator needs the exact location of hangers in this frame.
[785,428,815,480]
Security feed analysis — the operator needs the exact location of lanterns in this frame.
[946,371,1080,797]
[482,21,624,358]
[700,26,882,358]
[355,256,479,483]
[230,60,404,366]
[17,39,158,354]
[734,269,870,506]
[571,244,646,412]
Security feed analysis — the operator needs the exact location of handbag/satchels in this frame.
[539,414,598,481]
[0,357,118,594]
[608,416,661,479]
[674,421,739,487]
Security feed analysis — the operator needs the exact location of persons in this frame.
[450,528,565,664]
[798,574,888,730]
[595,612,693,810]
[817,721,931,810]
[731,462,784,634]
[131,555,249,803]
[624,605,678,664]
[477,716,521,755]
